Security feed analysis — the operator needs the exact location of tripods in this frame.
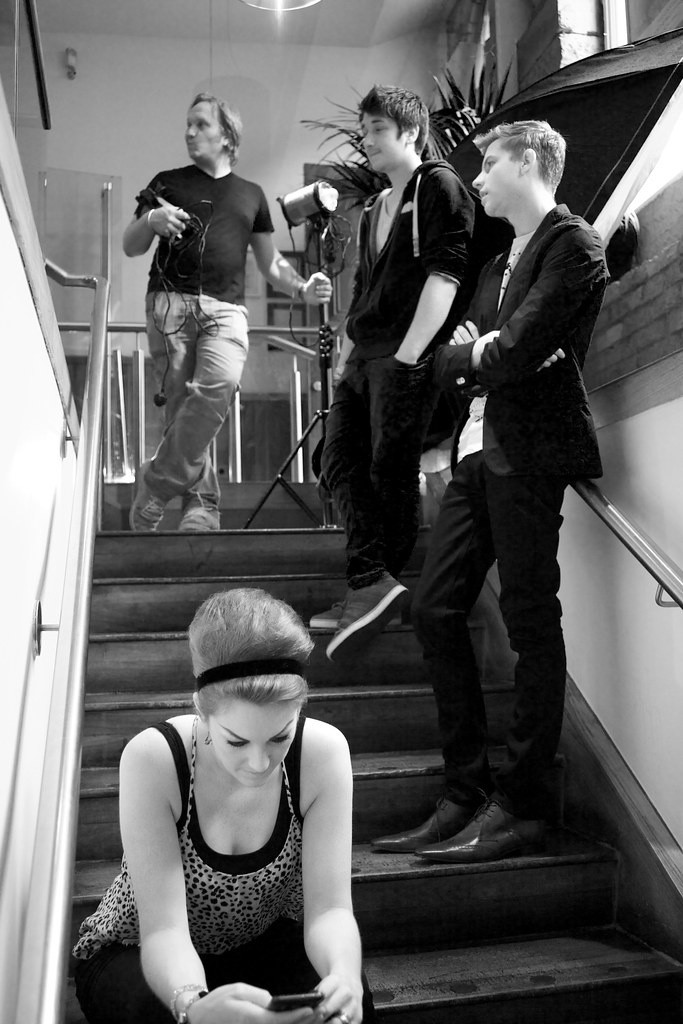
[244,218,338,534]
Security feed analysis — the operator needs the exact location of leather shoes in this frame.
[415,800,549,862]
[371,798,477,852]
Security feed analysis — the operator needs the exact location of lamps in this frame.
[65,48,76,80]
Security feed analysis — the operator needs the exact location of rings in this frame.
[338,1014,351,1024]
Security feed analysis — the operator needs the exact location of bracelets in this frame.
[170,984,209,1024]
[149,209,154,227]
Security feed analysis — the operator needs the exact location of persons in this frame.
[72,589,376,1024]
[369,120,612,863]
[309,85,476,659]
[120,93,332,531]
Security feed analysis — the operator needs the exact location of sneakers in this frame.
[129,461,165,531]
[310,598,402,628]
[326,571,410,663]
[179,492,219,530]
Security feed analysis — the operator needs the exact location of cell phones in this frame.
[268,992,325,1012]
